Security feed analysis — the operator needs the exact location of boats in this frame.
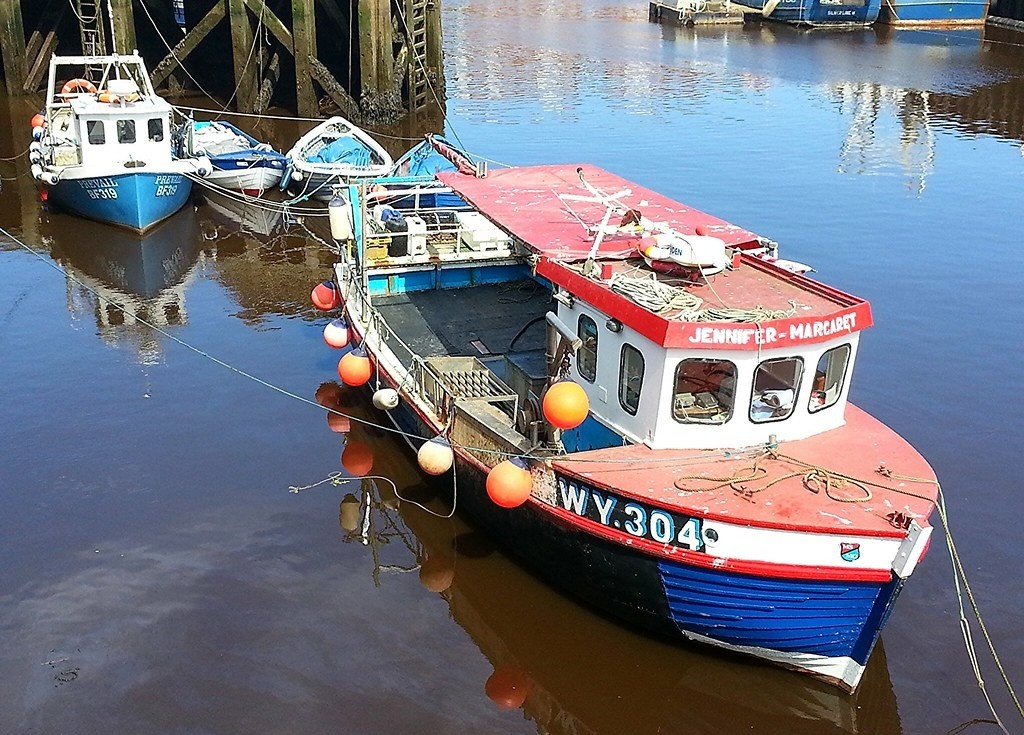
[310,137,942,696]
[731,0,882,27]
[313,378,904,734]
[178,121,288,197]
[40,204,203,328]
[30,5,194,236]
[648,0,745,28]
[200,186,286,237]
[390,133,478,177]
[287,116,395,203]
[876,1,990,26]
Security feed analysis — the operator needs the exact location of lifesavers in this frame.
[60,78,98,103]
[638,232,726,263]
[97,89,143,104]
[644,250,731,277]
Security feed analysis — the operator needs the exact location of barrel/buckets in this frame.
[381,209,403,222]
[374,205,394,220]
[405,216,426,254]
[385,218,407,256]
